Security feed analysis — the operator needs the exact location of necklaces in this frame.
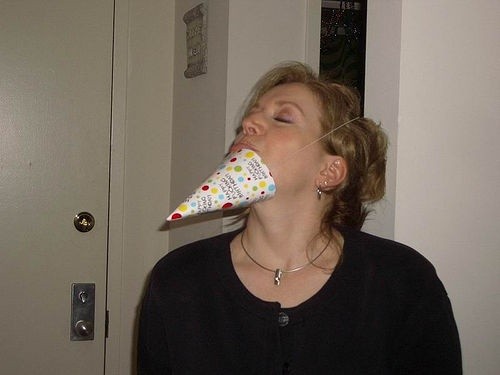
[241,228,332,286]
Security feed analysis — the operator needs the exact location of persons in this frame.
[132,59,462,375]
